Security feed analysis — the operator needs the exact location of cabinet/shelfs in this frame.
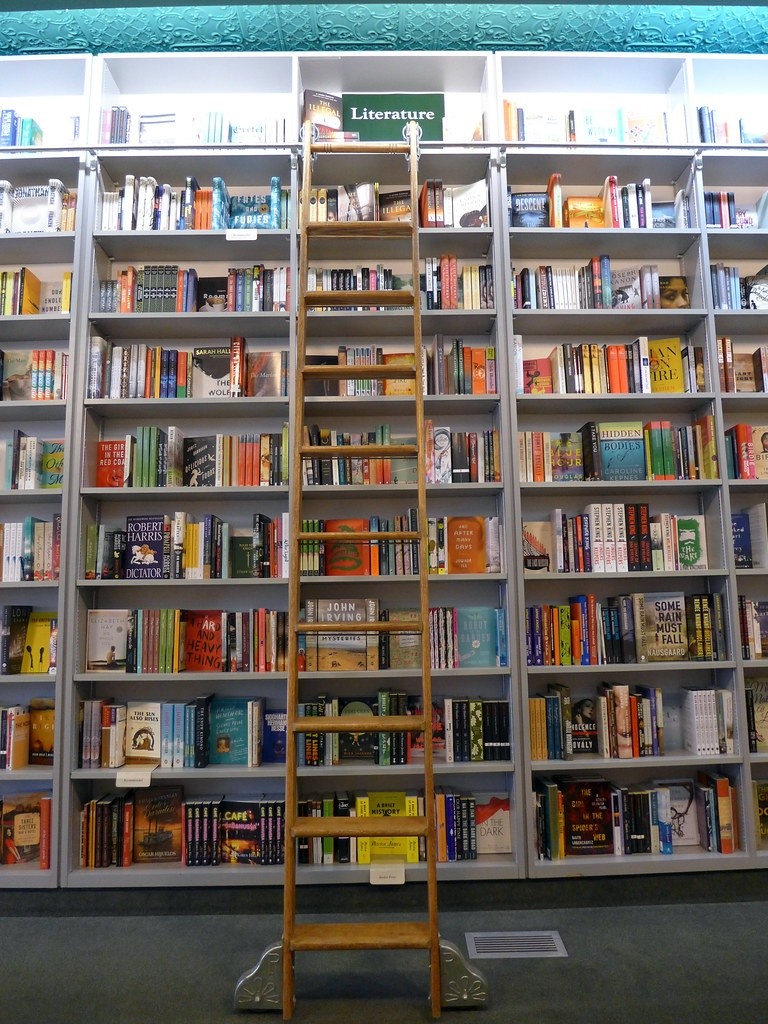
[0,51,768,888]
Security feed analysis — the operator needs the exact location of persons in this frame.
[572,699,597,738]
[659,276,691,309]
[106,646,118,667]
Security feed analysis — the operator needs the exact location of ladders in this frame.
[229,121,495,1024]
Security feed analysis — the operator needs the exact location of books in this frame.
[0,89,767,869]
